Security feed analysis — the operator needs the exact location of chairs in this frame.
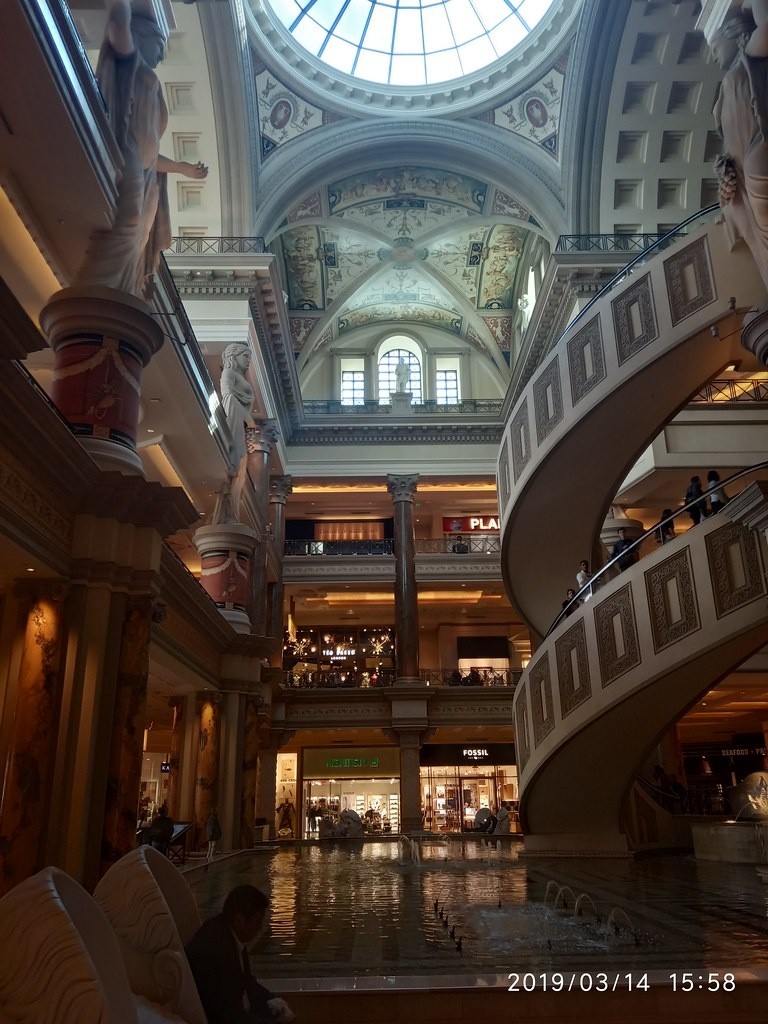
[0,844,208,1024]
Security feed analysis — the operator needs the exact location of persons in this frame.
[206,807,222,858]
[82,0,210,301]
[151,804,174,857]
[576,560,595,598]
[607,528,637,572]
[659,509,675,542]
[451,536,468,554]
[211,343,257,524]
[482,670,490,686]
[562,589,580,618]
[275,798,298,838]
[685,476,704,525]
[709,0,768,289]
[707,470,726,515]
[181,885,294,1024]
